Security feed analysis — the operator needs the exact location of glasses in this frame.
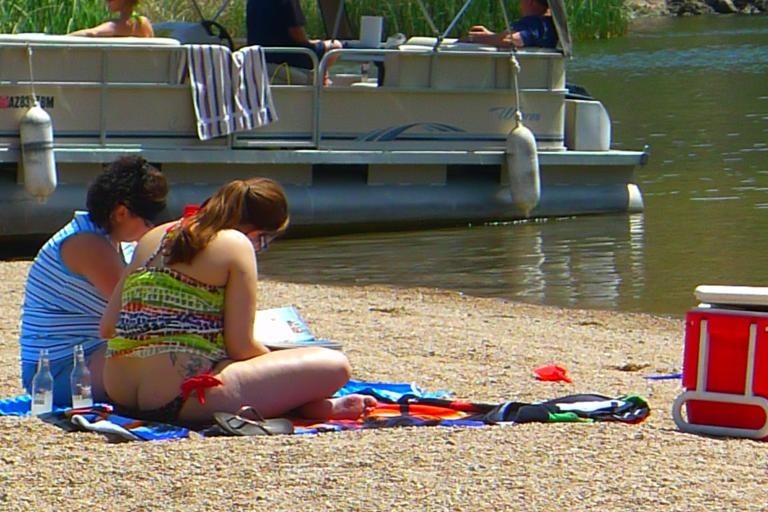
[255,235,269,254]
[119,199,158,228]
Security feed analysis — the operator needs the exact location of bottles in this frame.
[69,345,95,411]
[29,348,54,415]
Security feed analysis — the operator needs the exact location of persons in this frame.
[65,0,155,39]
[18,154,169,408]
[97,176,379,429]
[467,0,560,50]
[246,0,343,87]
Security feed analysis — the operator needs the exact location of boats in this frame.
[0,0,655,255]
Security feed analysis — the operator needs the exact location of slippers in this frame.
[210,404,294,436]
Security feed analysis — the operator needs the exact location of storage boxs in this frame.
[669,282,768,444]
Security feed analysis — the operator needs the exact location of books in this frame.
[251,305,343,350]
